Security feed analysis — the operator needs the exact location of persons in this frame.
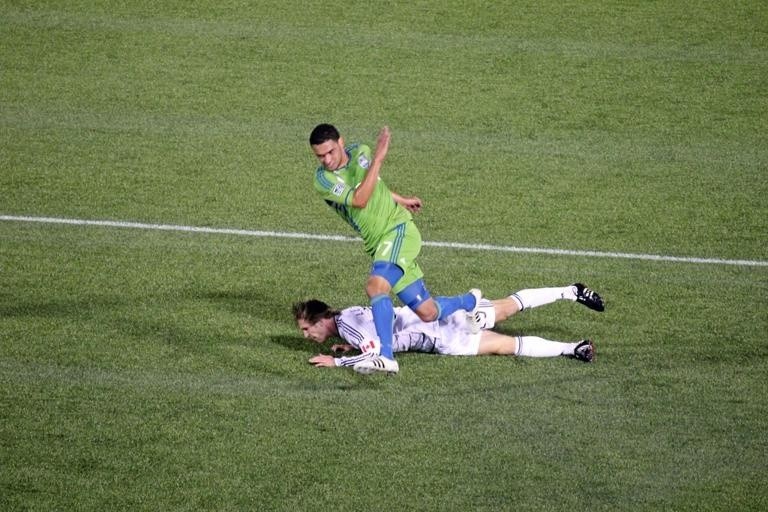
[293,282,604,369]
[309,123,481,377]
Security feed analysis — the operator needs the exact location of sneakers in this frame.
[464,286,485,335]
[352,355,399,376]
[571,281,605,313]
[573,339,595,363]
